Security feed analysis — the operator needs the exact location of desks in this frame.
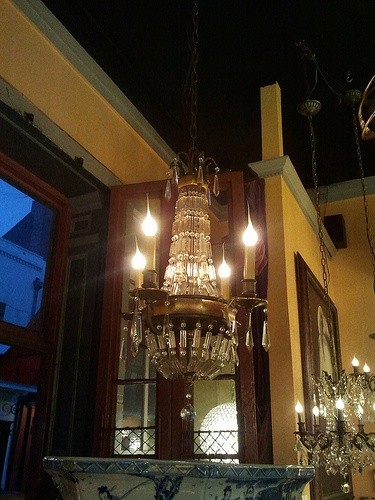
[42,456,315,500]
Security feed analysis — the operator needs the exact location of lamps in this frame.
[120,2,274,420]
[293,100,375,493]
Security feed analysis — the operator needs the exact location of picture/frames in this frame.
[294,250,356,500]
